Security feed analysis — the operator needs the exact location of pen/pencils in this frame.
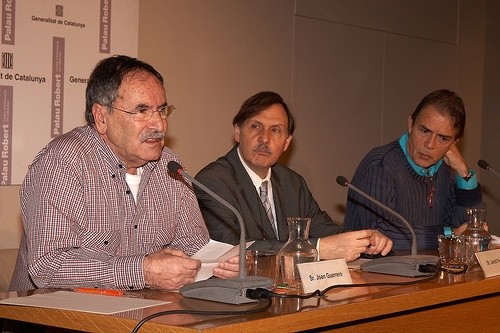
[74,288,127,297]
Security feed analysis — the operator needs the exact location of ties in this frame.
[259,181,278,240]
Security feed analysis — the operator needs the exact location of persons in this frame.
[10,54,254,298]
[192,91,394,263]
[342,89,483,250]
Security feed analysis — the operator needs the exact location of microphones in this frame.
[336,175,440,278]
[477,159,500,178]
[167,160,277,305]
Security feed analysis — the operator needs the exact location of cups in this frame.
[438,234,473,273]
[247,250,280,285]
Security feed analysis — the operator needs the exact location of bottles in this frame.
[461,208,491,272]
[279,217,318,290]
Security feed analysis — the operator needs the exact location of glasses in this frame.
[101,103,176,122]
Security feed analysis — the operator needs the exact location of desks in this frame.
[0,249,500,333]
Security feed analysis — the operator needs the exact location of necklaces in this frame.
[423,175,439,208]
